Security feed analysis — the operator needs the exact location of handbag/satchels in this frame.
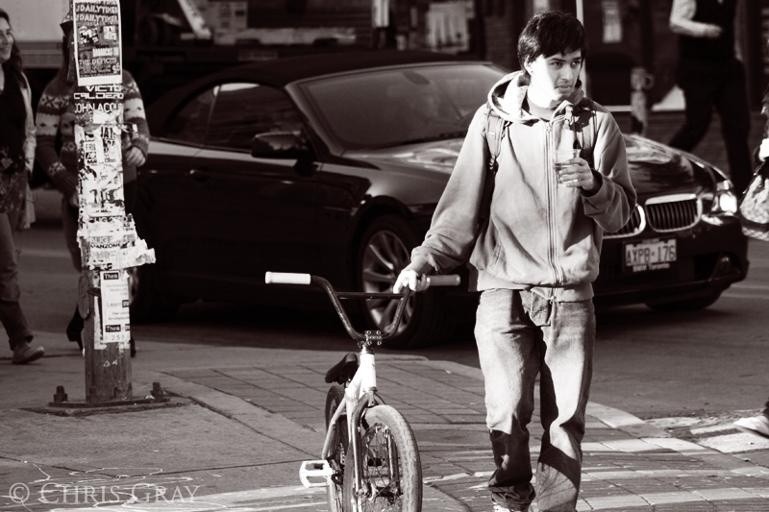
[739,173,769,241]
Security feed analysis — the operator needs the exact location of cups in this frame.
[553,149,581,188]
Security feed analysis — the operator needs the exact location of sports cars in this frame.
[57,55,751,332]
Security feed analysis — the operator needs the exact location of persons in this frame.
[34,8,151,357]
[664,2,754,192]
[2,8,47,364]
[392,7,637,512]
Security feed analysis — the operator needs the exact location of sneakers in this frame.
[488,494,536,512]
[13,342,45,364]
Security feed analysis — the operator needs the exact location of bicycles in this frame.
[261,265,463,510]
